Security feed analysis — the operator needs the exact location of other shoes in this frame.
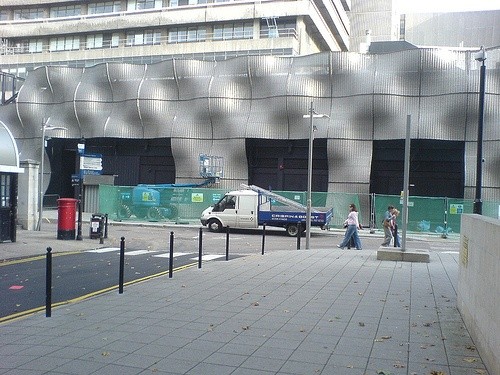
[353,247,362,250]
[337,245,344,250]
[351,246,356,248]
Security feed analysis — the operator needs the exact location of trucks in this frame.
[200,185,334,238]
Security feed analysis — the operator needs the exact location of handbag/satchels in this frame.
[383,219,391,227]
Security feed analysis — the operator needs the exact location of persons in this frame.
[391,209,400,247]
[381,205,396,247]
[337,204,362,249]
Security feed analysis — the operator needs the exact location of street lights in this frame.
[38,116,68,232]
[473,45,487,216]
[302,101,331,250]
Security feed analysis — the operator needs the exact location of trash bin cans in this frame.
[89,212,104,238]
